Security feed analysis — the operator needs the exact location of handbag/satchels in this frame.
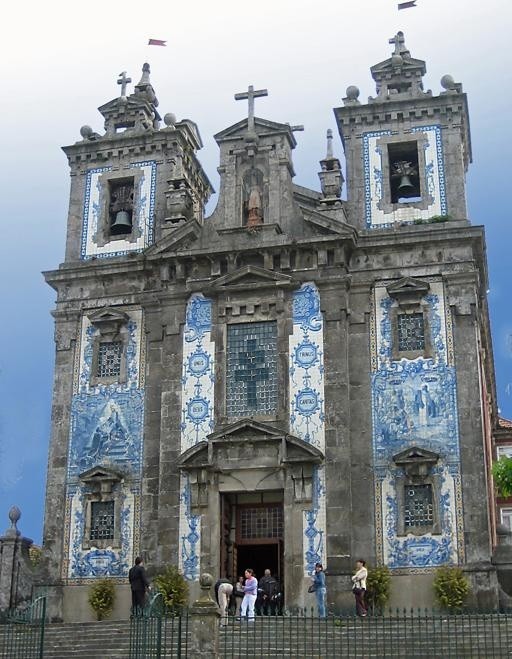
[308,585,316,593]
[353,577,361,594]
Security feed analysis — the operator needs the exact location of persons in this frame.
[311,562,327,620]
[244,173,263,218]
[128,555,152,619]
[234,568,282,625]
[213,577,238,628]
[351,559,368,617]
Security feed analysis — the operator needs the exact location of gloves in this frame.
[312,570,316,575]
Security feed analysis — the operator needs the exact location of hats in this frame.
[135,557,143,565]
